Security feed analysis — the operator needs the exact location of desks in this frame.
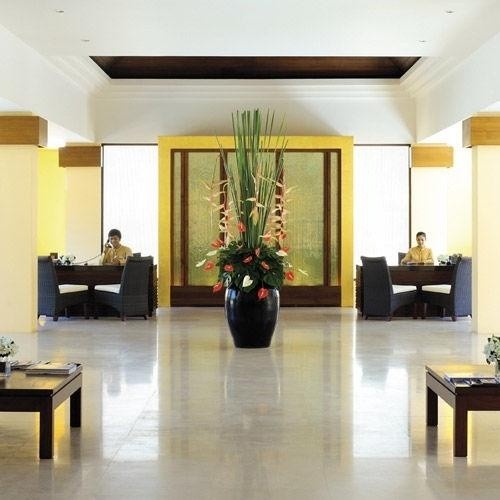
[45,264,159,317]
[356,264,467,318]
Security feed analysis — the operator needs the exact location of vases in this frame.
[0,358,13,372]
[222,286,282,348]
[491,363,500,376]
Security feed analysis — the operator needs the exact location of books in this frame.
[0,358,78,375]
[443,371,496,381]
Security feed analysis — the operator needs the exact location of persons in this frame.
[401,231,435,264]
[99,229,134,265]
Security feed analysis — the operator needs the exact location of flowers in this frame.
[195,107,309,302]
[484,336,500,365]
[0,336,16,358]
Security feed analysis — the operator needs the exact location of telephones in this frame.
[107,240,111,248]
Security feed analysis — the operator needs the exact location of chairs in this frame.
[398,252,406,263]
[133,253,141,261]
[38,256,89,322]
[94,256,151,321]
[361,256,419,323]
[422,256,472,322]
